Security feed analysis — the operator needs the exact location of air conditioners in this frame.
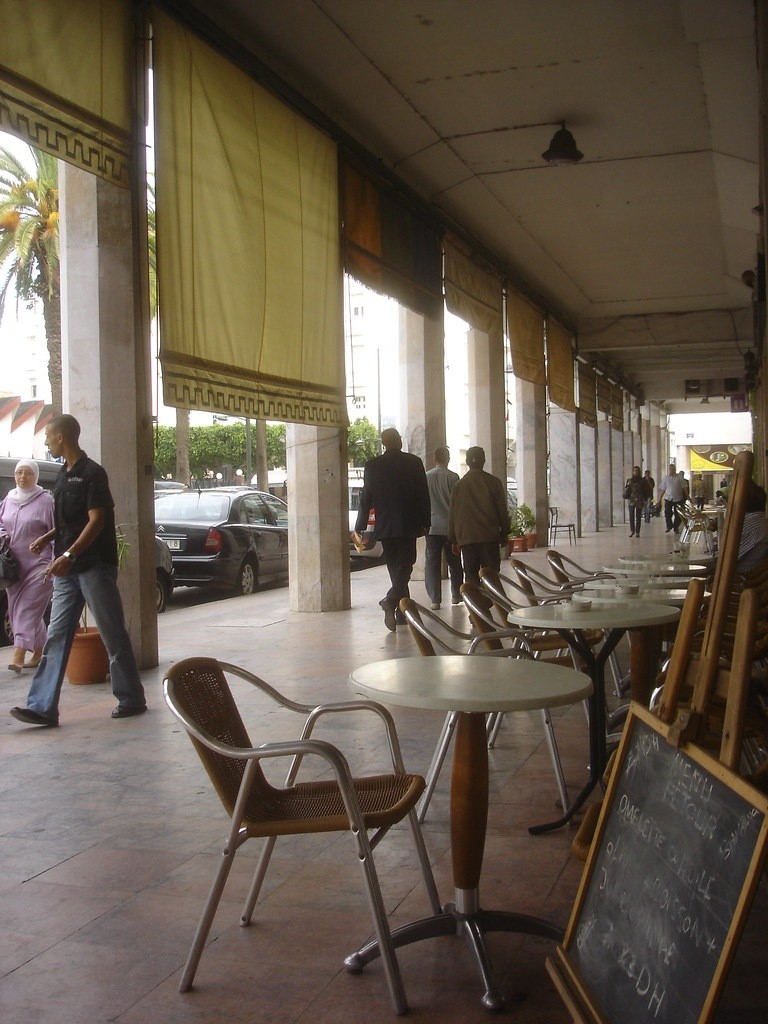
[684,380,709,399]
[722,378,745,398]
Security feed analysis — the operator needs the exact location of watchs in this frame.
[63,551,76,563]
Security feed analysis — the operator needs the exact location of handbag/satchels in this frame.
[0,538,18,590]
[623,486,630,499]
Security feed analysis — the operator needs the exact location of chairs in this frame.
[549,507,576,546]
[458,549,621,749]
[163,657,442,1015]
[676,503,718,554]
[398,598,591,826]
[527,556,768,863]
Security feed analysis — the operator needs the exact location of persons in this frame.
[11,413,148,726]
[0,457,54,672]
[625,463,728,538]
[450,446,513,626]
[703,479,768,586]
[355,427,431,633]
[423,446,466,610]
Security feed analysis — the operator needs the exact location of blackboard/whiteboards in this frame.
[542,701,768,1024]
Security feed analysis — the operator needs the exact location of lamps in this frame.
[541,121,584,166]
[701,398,710,404]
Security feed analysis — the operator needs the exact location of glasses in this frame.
[14,470,35,476]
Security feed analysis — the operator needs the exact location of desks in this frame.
[342,655,595,1011]
[506,552,709,772]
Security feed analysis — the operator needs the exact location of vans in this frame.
[346,468,385,566]
[0,458,176,648]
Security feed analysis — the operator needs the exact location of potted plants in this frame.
[517,503,539,549]
[507,505,517,556]
[64,521,135,685]
[509,503,528,552]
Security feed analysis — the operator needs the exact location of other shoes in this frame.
[8,663,22,673]
[637,533,639,537]
[10,707,59,724]
[674,528,679,534]
[629,532,634,537]
[453,595,464,604]
[379,597,396,632]
[431,602,439,609]
[396,608,407,625]
[665,527,672,532]
[112,698,147,718]
[23,652,41,668]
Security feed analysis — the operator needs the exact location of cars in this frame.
[152,480,186,498]
[149,486,289,598]
